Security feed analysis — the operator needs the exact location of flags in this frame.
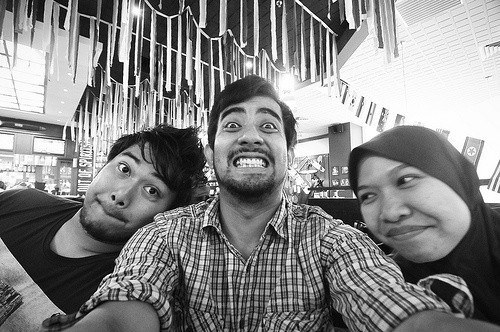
[460,136,500,194]
[336,79,350,104]
[414,121,450,140]
[355,97,405,133]
[347,88,358,112]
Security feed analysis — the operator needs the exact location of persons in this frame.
[0,122,209,332]
[38,76,500,332]
[15,183,28,189]
[348,126,500,326]
[0,181,7,192]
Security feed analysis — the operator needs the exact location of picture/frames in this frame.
[33,136,66,155]
[0,132,16,152]
